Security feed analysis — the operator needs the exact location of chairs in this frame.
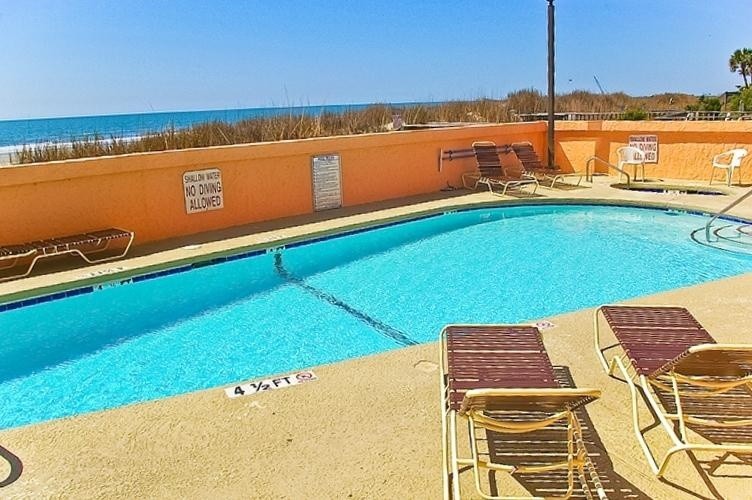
[510,141,584,188]
[616,145,647,182]
[435,320,610,499]
[593,302,752,482]
[460,140,539,198]
[709,147,748,187]
[0,225,136,283]
